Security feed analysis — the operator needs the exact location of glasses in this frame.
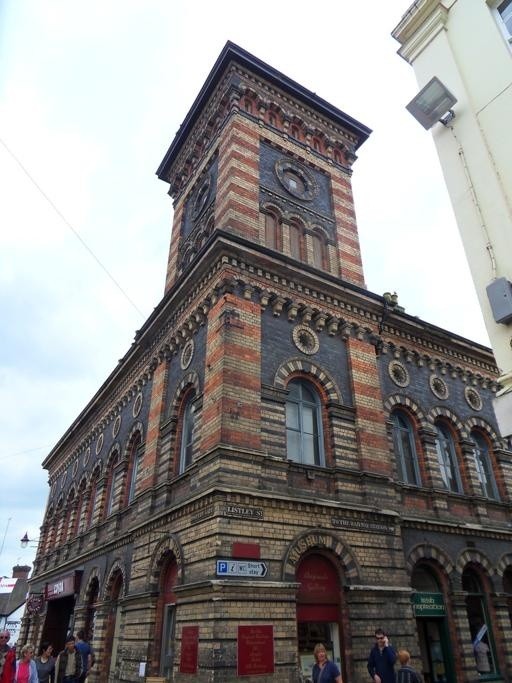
[376,637,384,640]
[0,636,8,639]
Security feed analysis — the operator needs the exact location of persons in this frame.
[367,628,397,683]
[475,642,492,672]
[312,643,342,682]
[394,650,423,682]
[0,630,94,683]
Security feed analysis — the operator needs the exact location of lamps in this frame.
[19,531,40,548]
[405,76,458,131]
